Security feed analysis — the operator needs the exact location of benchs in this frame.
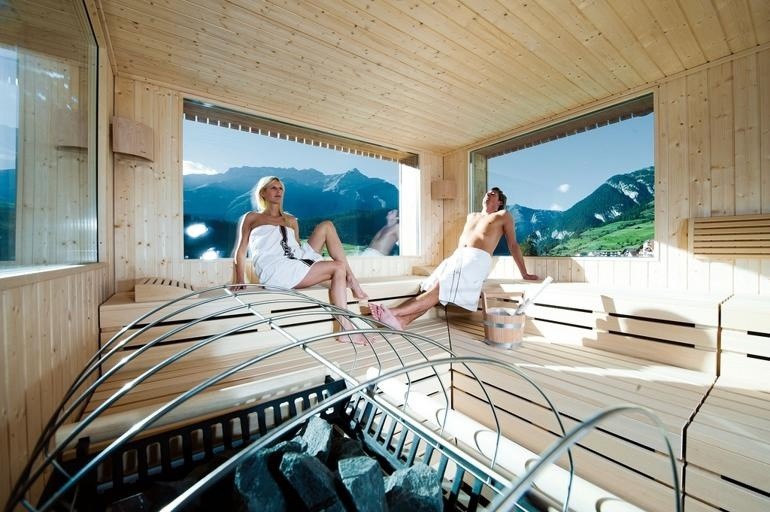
[99,272,770,400]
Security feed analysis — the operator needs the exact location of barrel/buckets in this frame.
[483,290,527,348]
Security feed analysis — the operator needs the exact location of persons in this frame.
[230,175,376,346]
[368,187,540,331]
[360,209,400,256]
